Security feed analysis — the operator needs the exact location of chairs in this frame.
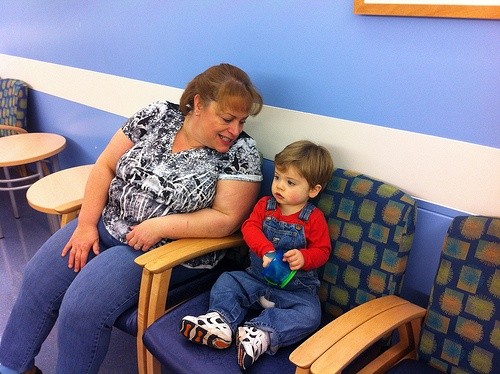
[311,215,500,374]
[0,78,28,176]
[55,198,241,374]
[145,167,416,374]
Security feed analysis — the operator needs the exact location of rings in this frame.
[134,244,140,251]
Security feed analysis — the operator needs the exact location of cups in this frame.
[262,251,297,288]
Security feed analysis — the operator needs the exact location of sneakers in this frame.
[237,327,268,371]
[179,312,232,349]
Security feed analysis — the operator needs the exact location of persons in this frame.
[0,63,264,374]
[180,139,334,373]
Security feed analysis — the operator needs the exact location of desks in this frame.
[26,164,94,223]
[0,133,67,239]
